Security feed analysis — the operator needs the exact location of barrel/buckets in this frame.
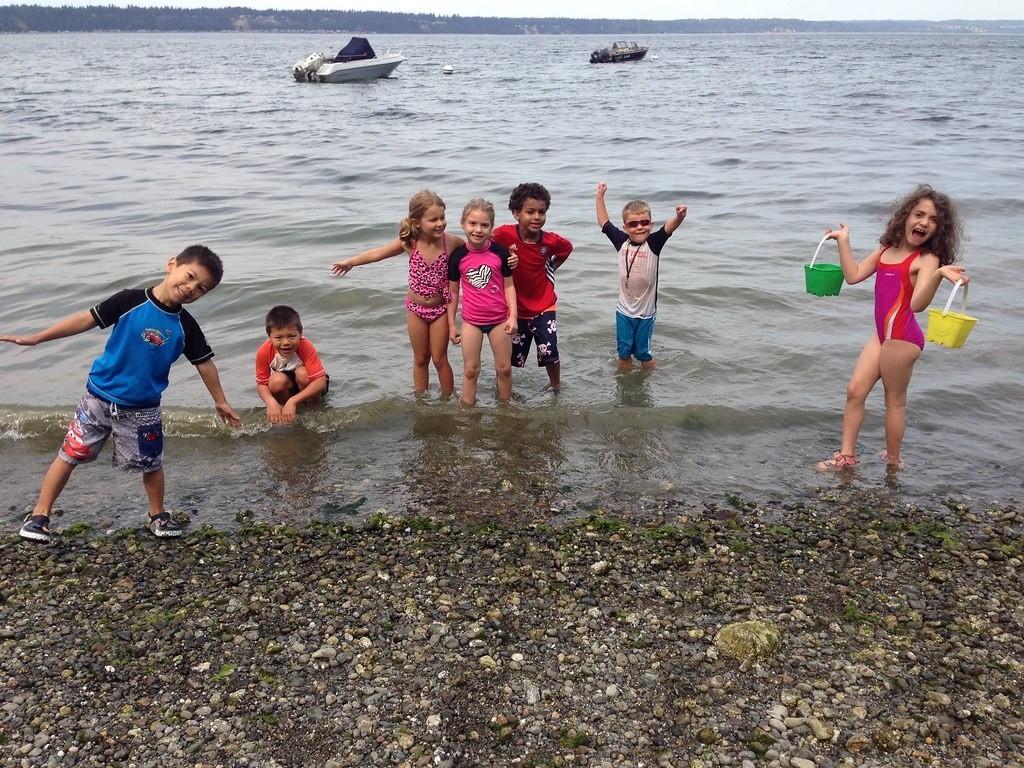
[804,233,847,299]
[926,279,977,349]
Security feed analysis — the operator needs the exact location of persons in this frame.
[256,306,329,424]
[816,184,968,472]
[448,199,518,404]
[492,182,573,391]
[0,245,243,545]
[595,182,688,370]
[331,191,518,395]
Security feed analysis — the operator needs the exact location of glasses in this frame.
[624,219,650,228]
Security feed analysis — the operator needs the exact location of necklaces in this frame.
[625,242,641,288]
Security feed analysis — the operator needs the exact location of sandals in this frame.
[816,450,857,474]
[881,450,903,469]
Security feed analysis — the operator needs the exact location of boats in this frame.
[290,36,407,85]
[590,40,651,65]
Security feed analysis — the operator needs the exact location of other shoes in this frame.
[146,510,181,536]
[20,512,50,544]
